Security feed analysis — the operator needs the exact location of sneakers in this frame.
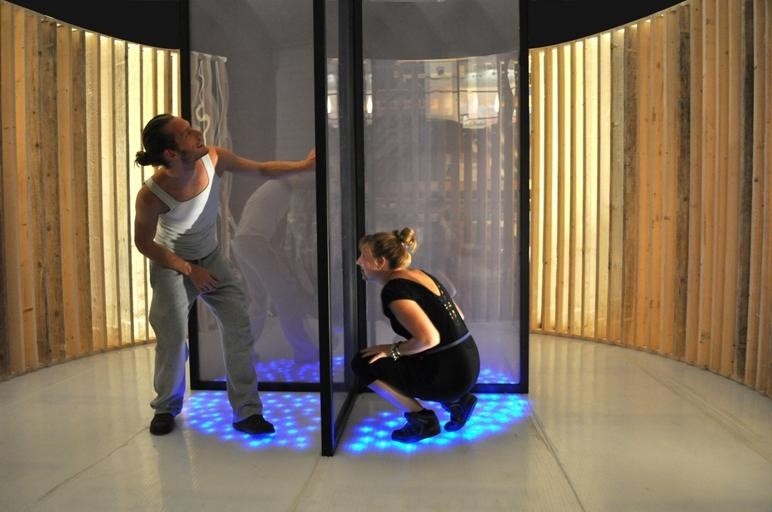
[150,412,174,436]
[442,392,478,431]
[233,415,274,434]
[391,407,441,444]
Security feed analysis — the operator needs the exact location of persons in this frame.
[233,172,319,366]
[133,112,315,435]
[352,224,482,444]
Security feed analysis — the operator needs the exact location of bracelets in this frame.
[390,341,403,361]
